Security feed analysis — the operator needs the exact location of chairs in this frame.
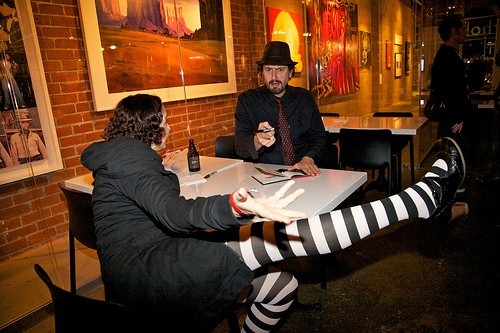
[34,111,416,333]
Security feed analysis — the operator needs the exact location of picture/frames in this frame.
[307,0,361,108]
[76,0,238,112]
[0,0,63,186]
[395,54,402,79]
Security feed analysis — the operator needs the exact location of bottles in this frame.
[187,139,200,173]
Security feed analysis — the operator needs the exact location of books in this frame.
[251,165,308,186]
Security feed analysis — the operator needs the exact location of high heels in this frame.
[420,136,468,227]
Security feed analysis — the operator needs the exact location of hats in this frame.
[256,41,298,65]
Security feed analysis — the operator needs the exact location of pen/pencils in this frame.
[254,126,275,133]
[203,171,217,179]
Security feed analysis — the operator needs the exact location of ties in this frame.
[276,98,295,167]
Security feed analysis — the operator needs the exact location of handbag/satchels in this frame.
[424,91,473,123]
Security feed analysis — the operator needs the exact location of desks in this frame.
[177,162,368,310]
[320,117,428,194]
[65,153,241,317]
[467,90,496,106]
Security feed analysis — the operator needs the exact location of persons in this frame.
[425,13,470,151]
[0,0,48,169]
[235,42,329,177]
[81,94,467,333]
